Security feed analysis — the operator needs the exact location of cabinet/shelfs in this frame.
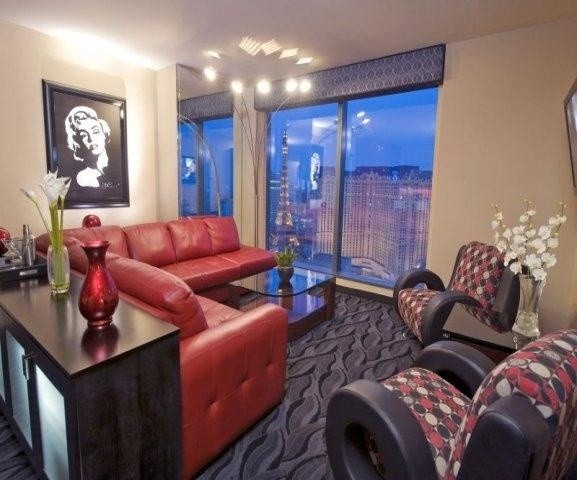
[0,304,182,480]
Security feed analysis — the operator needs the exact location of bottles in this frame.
[21,224,36,267]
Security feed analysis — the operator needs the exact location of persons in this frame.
[63,105,122,202]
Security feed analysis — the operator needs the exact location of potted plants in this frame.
[273,242,296,284]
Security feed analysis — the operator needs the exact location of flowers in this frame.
[19,170,74,290]
[487,199,569,324]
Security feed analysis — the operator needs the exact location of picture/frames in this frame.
[41,78,128,209]
[180,155,198,184]
[308,144,324,199]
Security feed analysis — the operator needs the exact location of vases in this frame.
[78,241,119,330]
[45,243,71,295]
